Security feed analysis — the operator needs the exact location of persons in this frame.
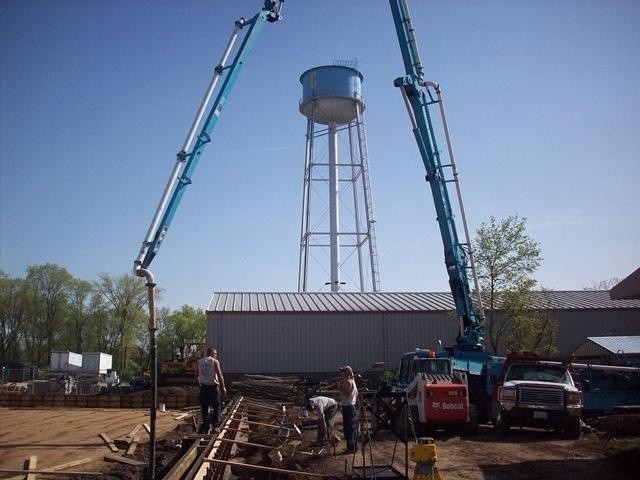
[367,381,388,431]
[335,365,358,456]
[305,395,338,445]
[192,347,228,433]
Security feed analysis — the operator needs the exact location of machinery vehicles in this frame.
[128,340,208,389]
[391,348,479,438]
[133,0,502,428]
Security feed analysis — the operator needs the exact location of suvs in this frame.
[495,360,586,439]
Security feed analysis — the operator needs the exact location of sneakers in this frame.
[339,447,357,455]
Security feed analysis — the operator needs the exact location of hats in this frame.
[339,366,353,372]
